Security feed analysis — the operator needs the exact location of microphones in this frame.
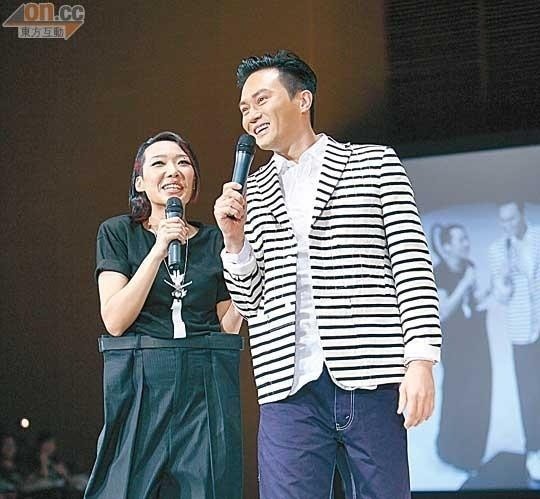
[165,196,187,272]
[227,133,257,220]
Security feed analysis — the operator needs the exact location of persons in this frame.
[428,223,493,474]
[83,128,246,499]
[484,196,540,483]
[24,429,70,499]
[0,431,24,499]
[214,49,442,499]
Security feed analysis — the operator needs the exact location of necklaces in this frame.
[146,214,193,301]
